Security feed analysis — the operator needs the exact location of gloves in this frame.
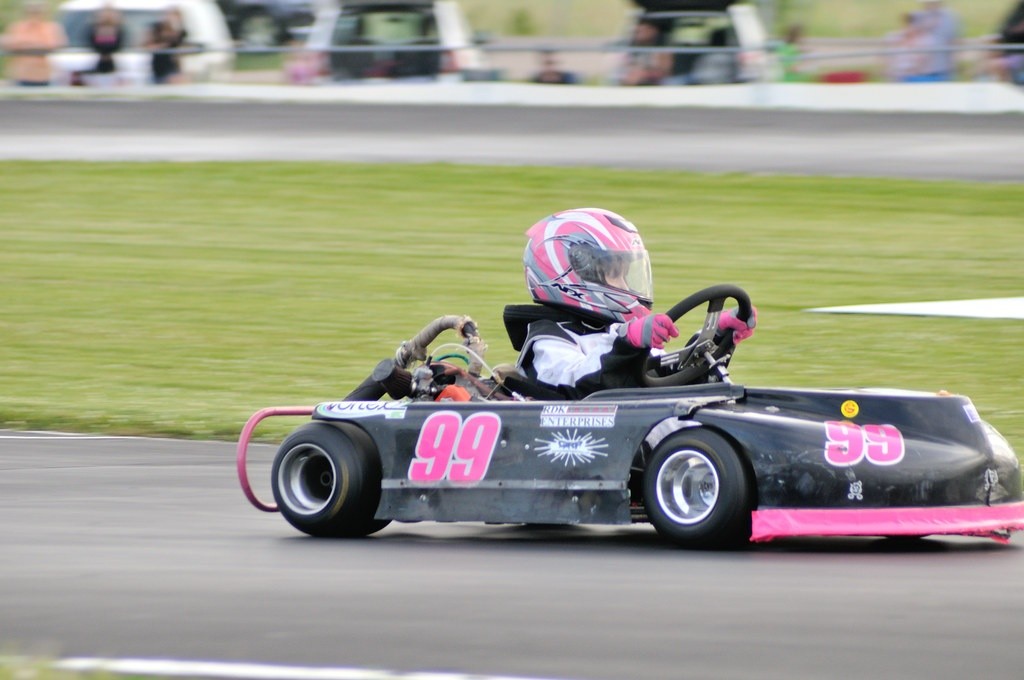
[718,306,758,344]
[627,313,679,349]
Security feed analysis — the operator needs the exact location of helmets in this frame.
[523,208,651,322]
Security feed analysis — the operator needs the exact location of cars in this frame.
[611,1,789,89]
[219,0,319,55]
[42,2,237,84]
[279,0,489,87]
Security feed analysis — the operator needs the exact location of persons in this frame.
[506,208,758,397]
[533,47,575,85]
[614,0,1022,87]
[290,1,470,84]
[0,3,188,86]
